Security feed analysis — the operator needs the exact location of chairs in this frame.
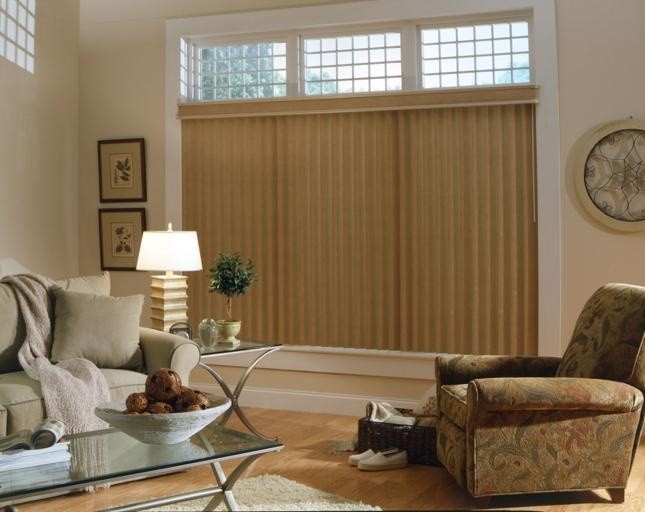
[435,282,645,509]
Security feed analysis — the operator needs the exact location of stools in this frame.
[413,381,438,428]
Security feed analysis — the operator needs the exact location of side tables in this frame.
[178,332,282,446]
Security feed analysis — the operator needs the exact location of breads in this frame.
[125,367,211,415]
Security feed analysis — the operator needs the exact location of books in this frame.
[1,417,73,475]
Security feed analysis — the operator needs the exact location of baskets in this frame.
[357,406,445,467]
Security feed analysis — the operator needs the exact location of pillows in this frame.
[49,283,147,375]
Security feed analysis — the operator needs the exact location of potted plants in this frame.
[207,248,259,347]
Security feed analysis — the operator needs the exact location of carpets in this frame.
[81,472,382,512]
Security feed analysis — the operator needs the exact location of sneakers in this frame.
[347,446,409,472]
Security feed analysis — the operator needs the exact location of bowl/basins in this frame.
[94,390,232,445]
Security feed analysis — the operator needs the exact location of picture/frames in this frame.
[97,208,146,272]
[97,137,147,203]
[574,118,645,233]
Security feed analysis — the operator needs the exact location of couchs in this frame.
[0,272,200,471]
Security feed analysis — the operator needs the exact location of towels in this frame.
[329,400,416,454]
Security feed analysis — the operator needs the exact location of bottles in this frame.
[198,318,217,348]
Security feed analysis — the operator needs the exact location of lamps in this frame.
[135,222,204,335]
